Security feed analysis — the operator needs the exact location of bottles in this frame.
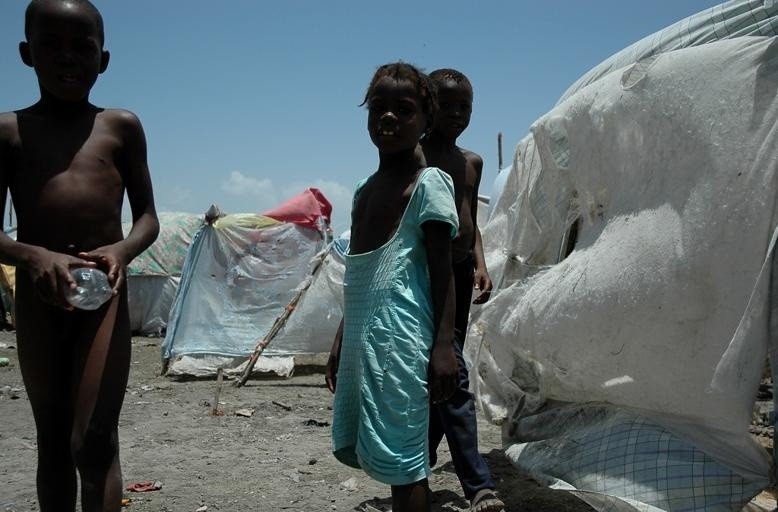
[60,265,114,311]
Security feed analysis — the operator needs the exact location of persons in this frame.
[322,61,463,512]
[420,67,509,512]
[0,1,159,512]
